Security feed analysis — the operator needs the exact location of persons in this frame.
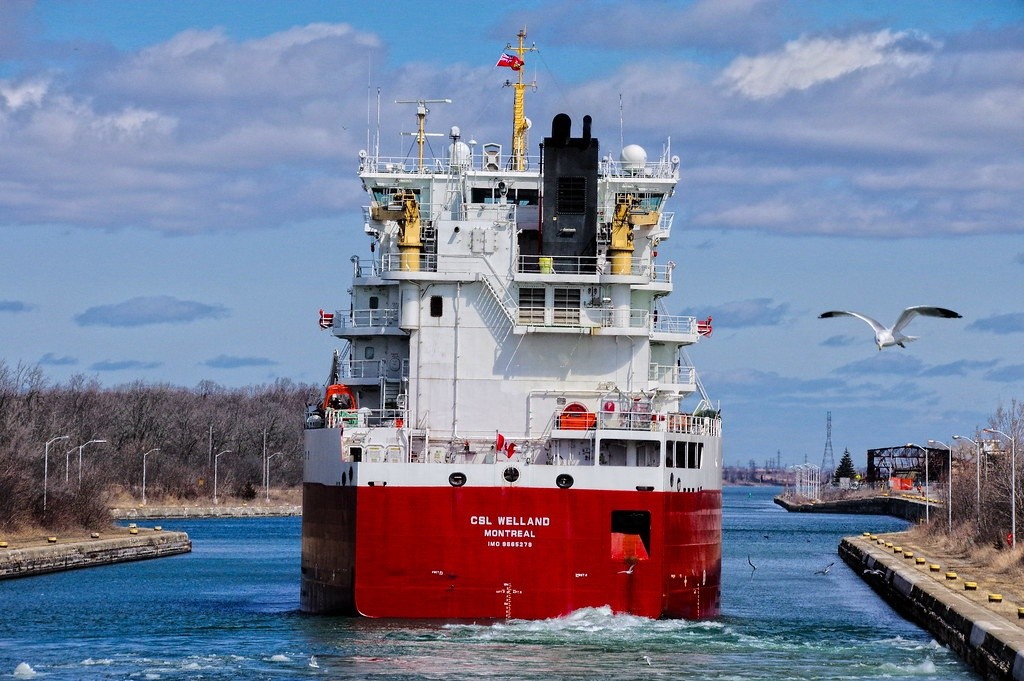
[1007,529,1016,548]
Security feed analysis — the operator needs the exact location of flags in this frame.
[496,434,518,458]
[496,52,525,70]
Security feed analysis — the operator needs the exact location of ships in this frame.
[300,30,720,620]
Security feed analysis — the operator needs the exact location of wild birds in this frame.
[743,534,885,582]
[818,306,963,352]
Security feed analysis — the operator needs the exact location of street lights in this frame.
[66,446,80,483]
[926,439,952,529]
[981,427,1016,549]
[951,434,980,533]
[215,450,232,504]
[43,435,70,510]
[904,442,929,525]
[79,438,107,490]
[267,452,284,500]
[143,448,162,501]
